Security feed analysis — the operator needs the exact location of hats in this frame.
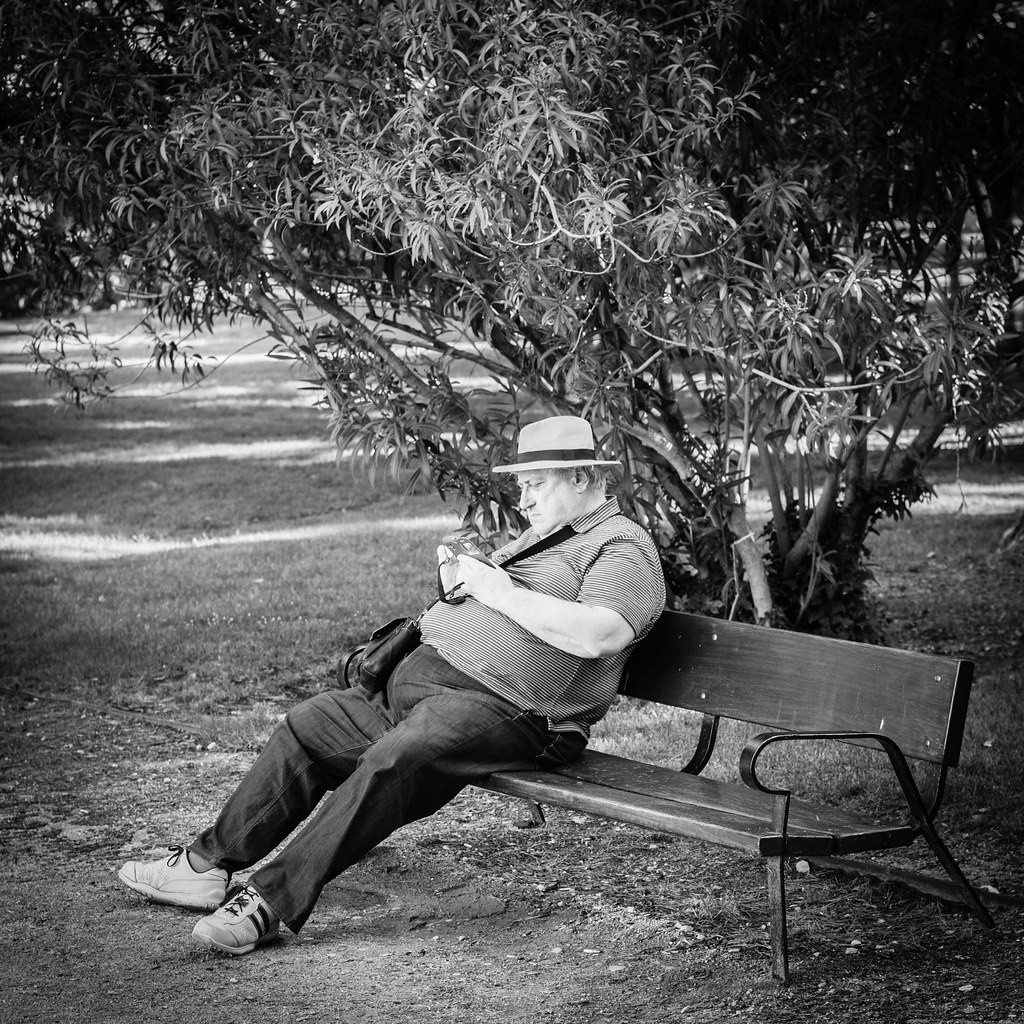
[492,415,621,473]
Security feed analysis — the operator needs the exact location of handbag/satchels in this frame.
[360,616,423,692]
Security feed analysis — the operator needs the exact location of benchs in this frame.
[337,610,994,983]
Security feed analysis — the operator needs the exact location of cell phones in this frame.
[444,536,497,571]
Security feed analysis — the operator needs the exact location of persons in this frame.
[116,414,666,957]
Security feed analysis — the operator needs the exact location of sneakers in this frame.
[118,844,229,909]
[192,884,282,954]
